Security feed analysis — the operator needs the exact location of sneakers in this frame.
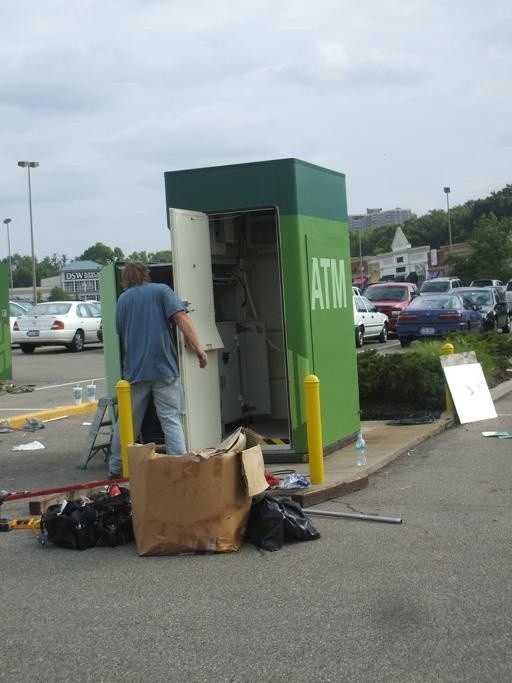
[108,472,122,479]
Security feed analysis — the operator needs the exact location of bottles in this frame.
[355,432,368,467]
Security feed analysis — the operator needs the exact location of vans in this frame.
[419,275,463,296]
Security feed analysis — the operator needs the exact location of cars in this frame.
[468,278,503,286]
[353,294,390,347]
[8,296,103,353]
[362,281,420,338]
[352,286,363,296]
[446,286,510,335]
[498,278,511,316]
[395,294,485,348]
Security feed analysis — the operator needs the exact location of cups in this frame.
[86,383,97,402]
[72,386,83,404]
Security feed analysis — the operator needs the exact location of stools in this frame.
[76,398,145,470]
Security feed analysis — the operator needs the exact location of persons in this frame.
[105,259,208,478]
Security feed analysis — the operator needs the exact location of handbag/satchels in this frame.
[40,481,131,549]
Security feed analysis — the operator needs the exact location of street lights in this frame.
[443,186,453,253]
[353,214,364,290]
[4,216,13,299]
[19,159,39,304]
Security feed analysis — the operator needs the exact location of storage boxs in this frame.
[123,425,268,556]
